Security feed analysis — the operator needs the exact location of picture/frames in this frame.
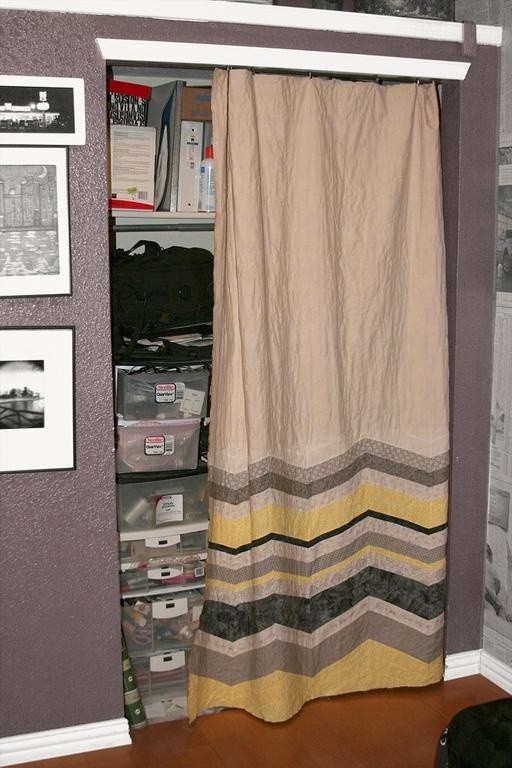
[0,73,87,147]
[0,325,76,475]
[0,146,72,298]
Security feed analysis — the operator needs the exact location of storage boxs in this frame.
[112,363,213,420]
[107,123,159,214]
[114,465,211,529]
[114,413,202,470]
[126,650,189,723]
[183,85,215,122]
[120,590,202,651]
[118,528,208,591]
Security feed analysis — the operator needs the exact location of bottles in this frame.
[198,144,216,211]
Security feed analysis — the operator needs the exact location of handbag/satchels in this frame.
[110,239,221,333]
